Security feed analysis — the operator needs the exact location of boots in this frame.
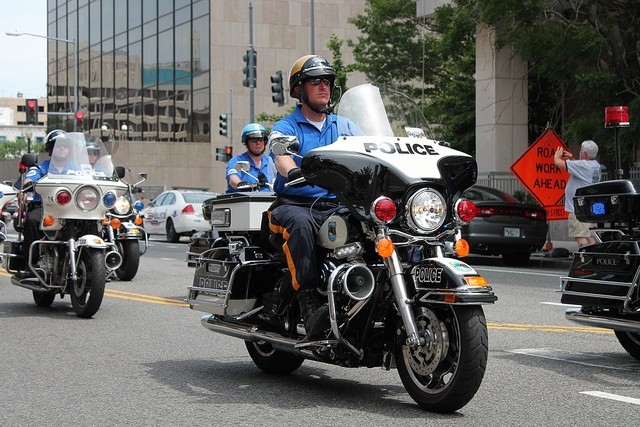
[300,294,330,340]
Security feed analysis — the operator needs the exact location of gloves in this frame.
[236,181,253,192]
[23,181,37,192]
[288,168,310,188]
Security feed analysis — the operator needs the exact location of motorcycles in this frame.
[187,159,276,267]
[94,154,147,281]
[555,105,639,363]
[0,131,128,318]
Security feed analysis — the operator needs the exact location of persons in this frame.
[82,138,101,165]
[22,129,81,282]
[554,140,601,247]
[226,123,278,192]
[267,54,362,340]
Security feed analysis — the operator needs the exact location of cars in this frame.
[5,196,34,211]
[448,186,549,267]
[139,189,221,243]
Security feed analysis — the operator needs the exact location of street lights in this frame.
[4,28,80,134]
[24,128,33,152]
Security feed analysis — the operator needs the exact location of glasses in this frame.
[248,138,263,142]
[84,150,98,156]
[53,142,68,148]
[304,76,332,86]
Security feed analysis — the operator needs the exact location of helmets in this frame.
[80,141,100,163]
[240,123,269,156]
[45,129,72,160]
[289,54,337,113]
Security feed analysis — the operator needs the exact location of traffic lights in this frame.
[25,98,38,125]
[74,110,84,132]
[270,70,284,106]
[219,114,227,136]
[216,147,225,161]
[185,82,498,412]
[242,50,257,87]
[225,145,232,161]
